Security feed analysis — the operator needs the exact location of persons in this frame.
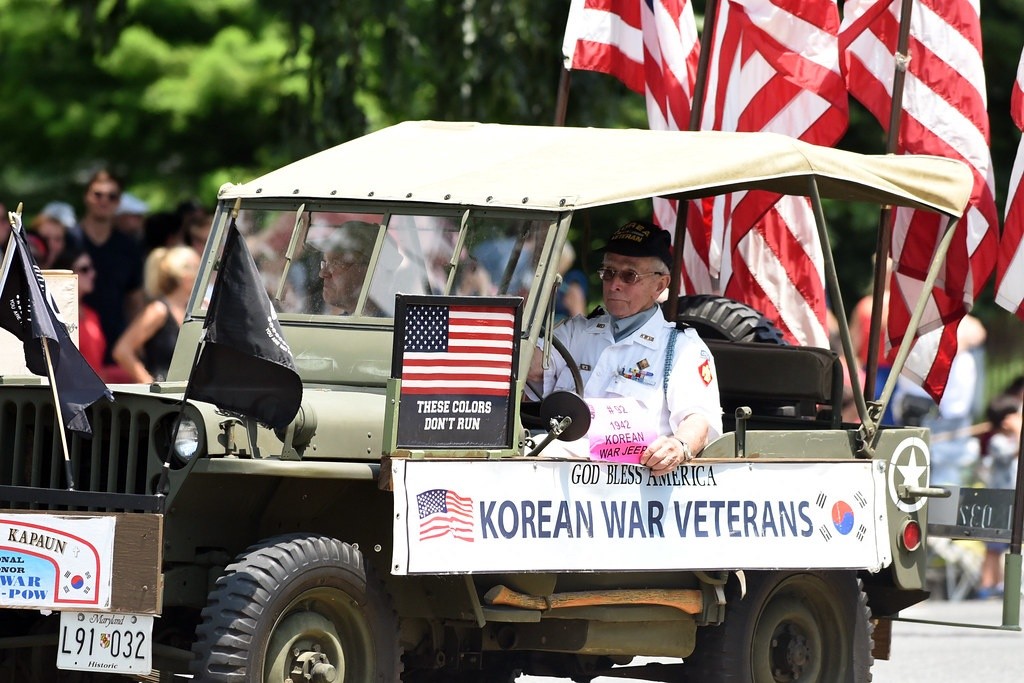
[848,252,1024,600]
[302,221,403,319]
[527,219,723,477]
[977,377,1024,600]
[0,169,284,384]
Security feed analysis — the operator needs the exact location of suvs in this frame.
[0,119,976,683]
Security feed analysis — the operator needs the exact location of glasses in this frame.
[597,266,665,285]
[76,262,94,273]
[90,191,117,203]
[319,258,365,271]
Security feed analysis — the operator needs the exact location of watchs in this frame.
[671,433,693,461]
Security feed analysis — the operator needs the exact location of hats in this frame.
[308,220,403,272]
[599,217,672,259]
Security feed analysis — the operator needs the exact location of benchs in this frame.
[705,336,843,431]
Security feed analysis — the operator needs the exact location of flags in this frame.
[0,226,115,430]
[561,0,1024,404]
[187,225,303,431]
